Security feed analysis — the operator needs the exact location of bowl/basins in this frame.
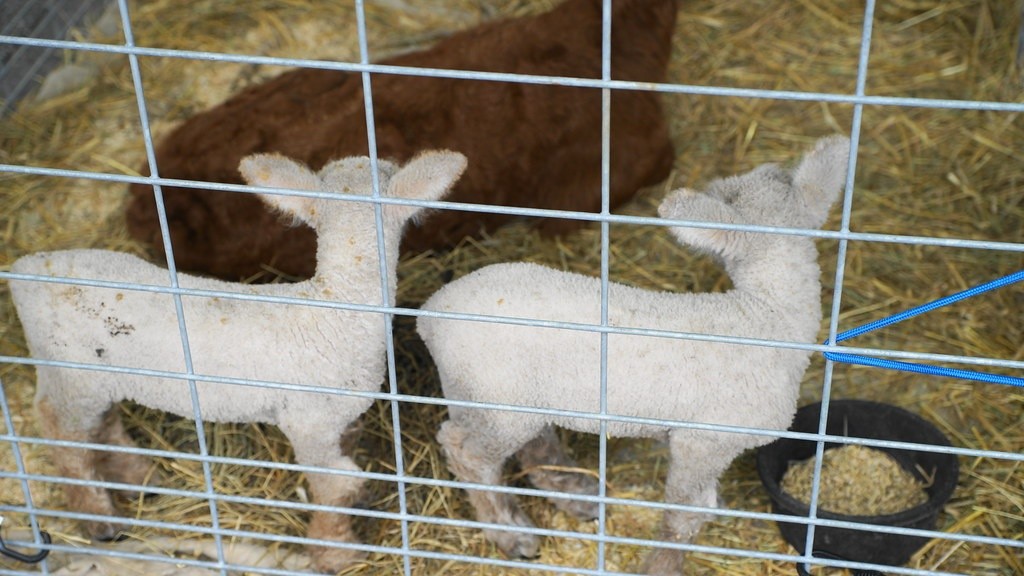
[756,398,957,574]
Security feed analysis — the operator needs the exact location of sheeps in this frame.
[8,149,468,572]
[122,0,679,298]
[414,132,850,576]
